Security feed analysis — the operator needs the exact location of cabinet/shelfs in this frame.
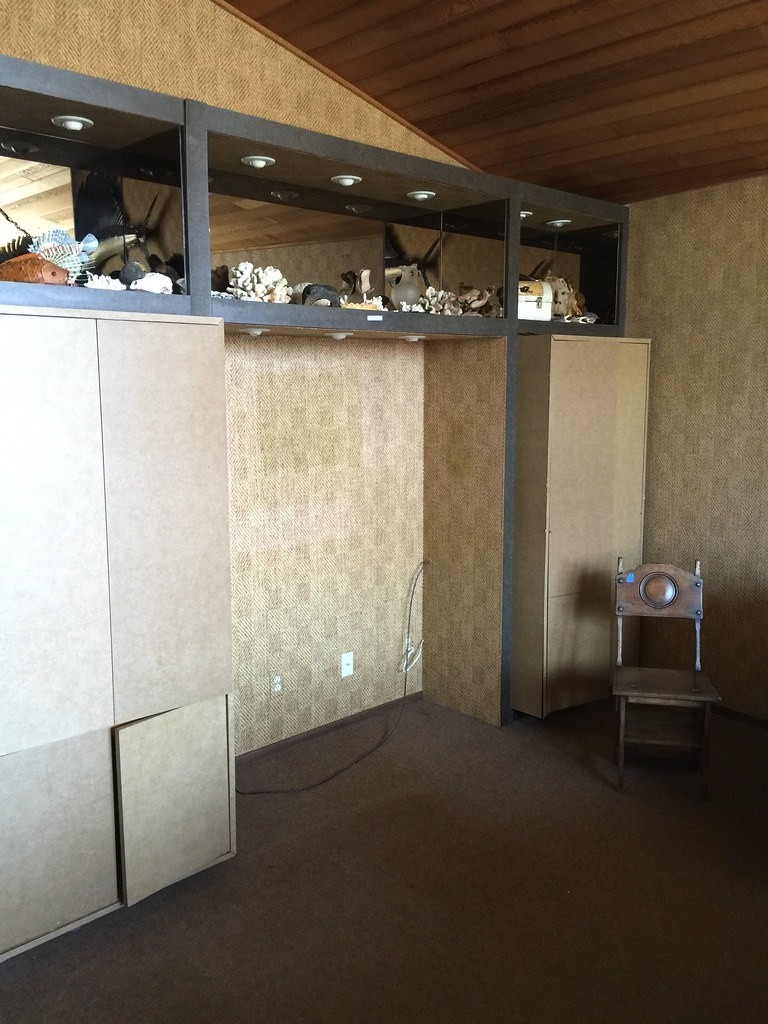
[0,53,653,974]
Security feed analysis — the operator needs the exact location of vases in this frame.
[390,263,420,312]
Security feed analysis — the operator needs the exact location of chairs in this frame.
[610,557,725,794]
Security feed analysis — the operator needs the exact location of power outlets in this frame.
[402,633,413,655]
[269,669,285,698]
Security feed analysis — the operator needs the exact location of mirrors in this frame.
[0,123,592,329]
